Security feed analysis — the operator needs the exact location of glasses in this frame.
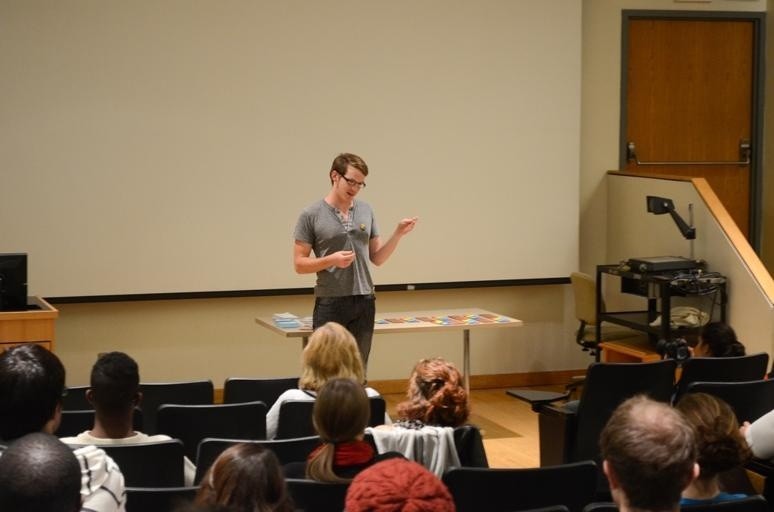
[334,169,366,189]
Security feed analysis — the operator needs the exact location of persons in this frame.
[396,357,471,431]
[58,352,197,486]
[597,320,774,512]
[266,320,393,440]
[0,433,96,512]
[294,154,419,385]
[0,344,127,512]
[190,443,299,512]
[281,378,408,482]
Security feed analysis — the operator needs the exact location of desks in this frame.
[255,306,523,406]
[595,264,727,365]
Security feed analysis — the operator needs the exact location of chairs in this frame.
[572,271,626,354]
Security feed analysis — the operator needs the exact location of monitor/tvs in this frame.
[0,252,28,312]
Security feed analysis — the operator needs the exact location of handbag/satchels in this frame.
[650,306,710,330]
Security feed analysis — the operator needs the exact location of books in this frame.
[272,311,301,329]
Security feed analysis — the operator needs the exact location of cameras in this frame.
[656,338,692,362]
